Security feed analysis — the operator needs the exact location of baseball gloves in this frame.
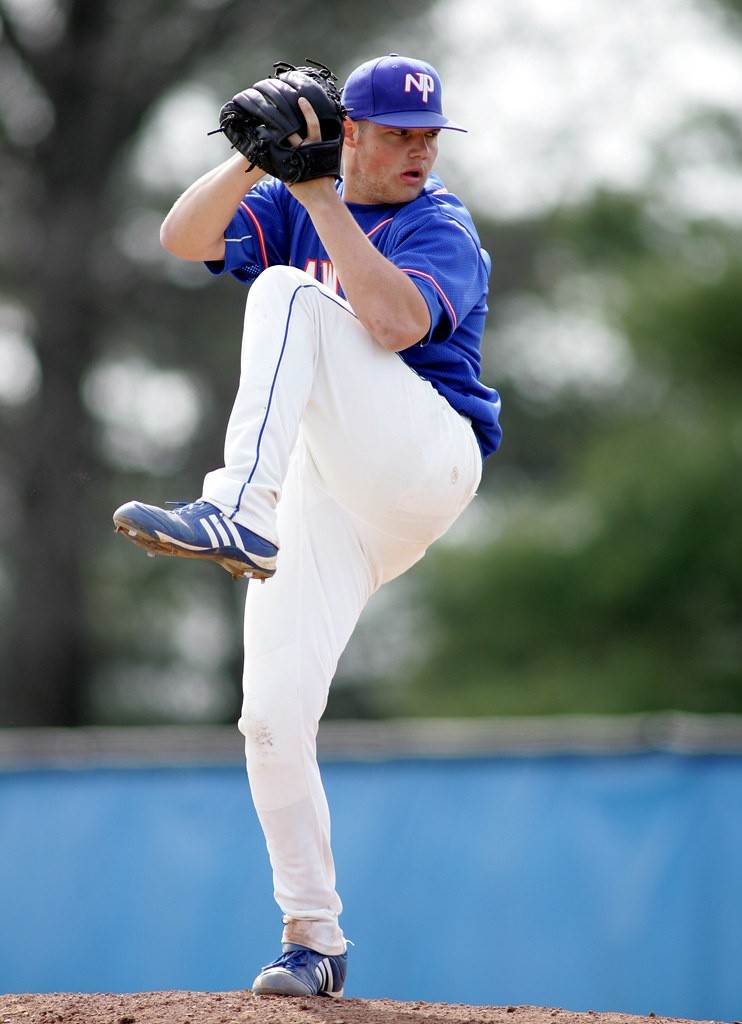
[203,57,350,190]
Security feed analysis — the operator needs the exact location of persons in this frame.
[105,55,505,996]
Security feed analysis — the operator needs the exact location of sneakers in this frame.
[113,499,278,584]
[253,943,348,998]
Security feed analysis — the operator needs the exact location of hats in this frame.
[340,53,464,132]
